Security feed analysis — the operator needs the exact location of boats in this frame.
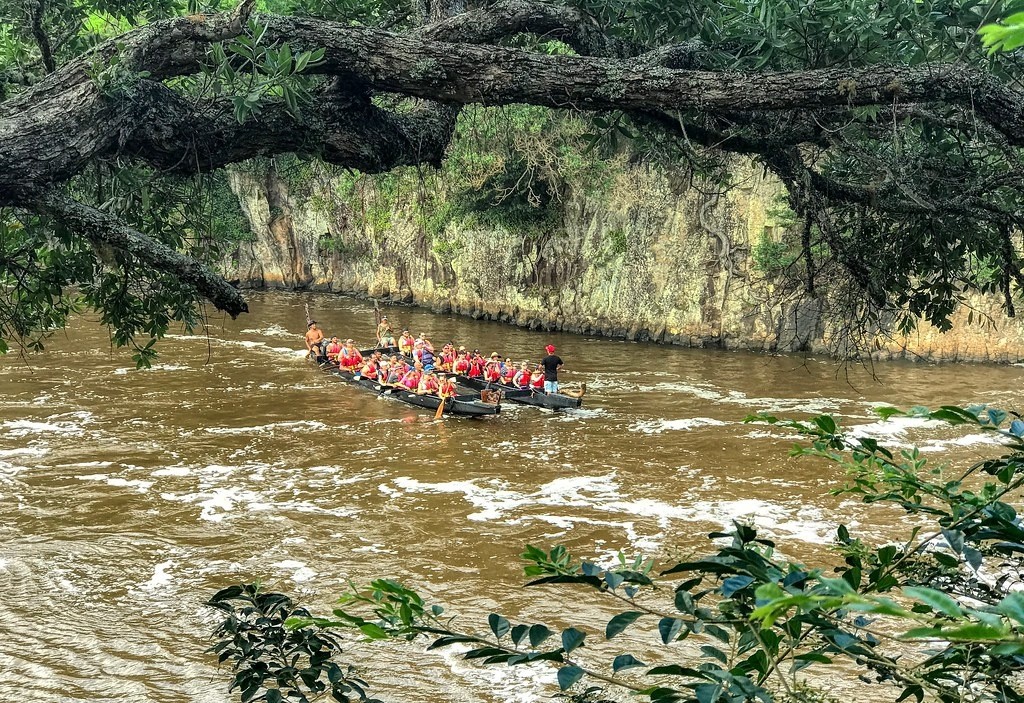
[375,339,583,410]
[309,338,501,416]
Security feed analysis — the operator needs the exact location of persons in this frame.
[326,335,457,400]
[305,320,323,356]
[538,344,564,396]
[398,327,545,390]
[377,315,398,349]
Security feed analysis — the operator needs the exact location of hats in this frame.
[402,326,409,332]
[459,346,465,351]
[546,345,555,353]
[346,339,353,344]
[424,364,435,371]
[485,358,497,367]
[307,320,317,327]
[331,334,336,340]
[397,355,404,359]
[447,340,453,343]
[382,315,388,321]
[491,351,502,358]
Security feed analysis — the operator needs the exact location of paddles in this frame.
[306,326,496,420]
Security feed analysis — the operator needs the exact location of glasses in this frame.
[440,380,444,382]
[444,348,449,349]
[449,344,453,345]
[473,358,478,360]
[420,335,426,337]
[522,364,528,366]
[506,360,512,362]
